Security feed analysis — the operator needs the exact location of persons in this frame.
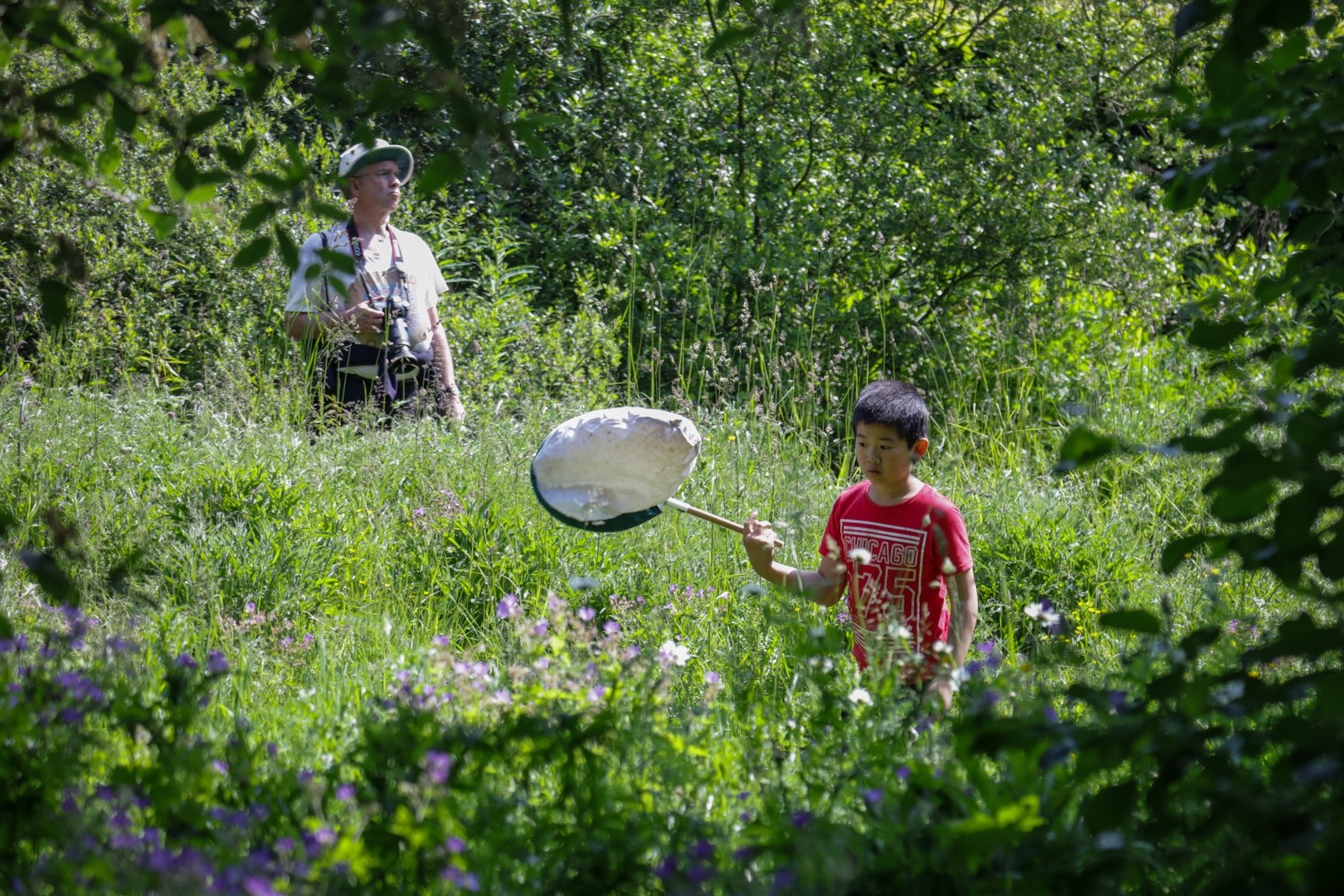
[743,379,977,720]
[284,139,467,446]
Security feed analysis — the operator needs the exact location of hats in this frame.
[338,137,414,188]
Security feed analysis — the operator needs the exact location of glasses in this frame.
[349,168,402,181]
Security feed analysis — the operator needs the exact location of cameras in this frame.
[369,294,417,374]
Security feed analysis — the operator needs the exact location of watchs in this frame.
[435,383,459,404]
